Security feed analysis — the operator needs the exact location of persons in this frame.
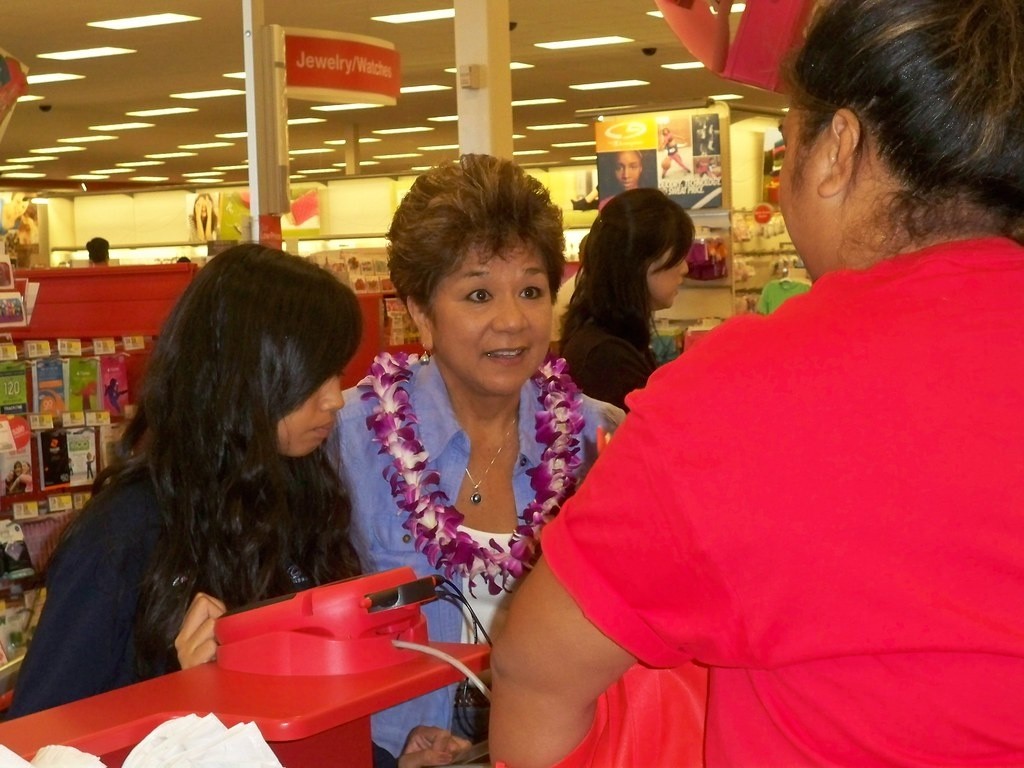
[176,256,191,265]
[694,116,716,179]
[487,0,1024,768]
[191,192,219,243]
[599,151,644,211]
[0,191,38,244]
[85,237,109,264]
[3,460,32,494]
[560,188,695,415]
[659,128,691,179]
[14,245,474,761]
[314,150,624,768]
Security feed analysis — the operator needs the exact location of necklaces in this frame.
[357,343,586,602]
[464,411,517,505]
